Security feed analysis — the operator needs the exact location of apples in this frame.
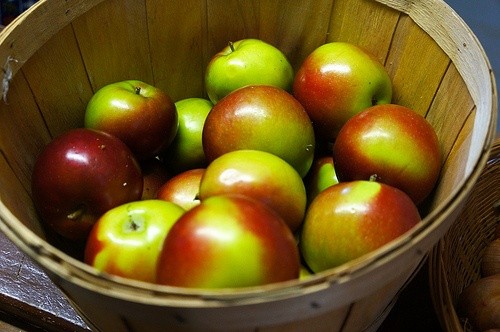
[33,40,439,290]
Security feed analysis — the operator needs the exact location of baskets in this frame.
[430,141,500,332]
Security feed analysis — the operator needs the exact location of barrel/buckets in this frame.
[1,0,500,331]
